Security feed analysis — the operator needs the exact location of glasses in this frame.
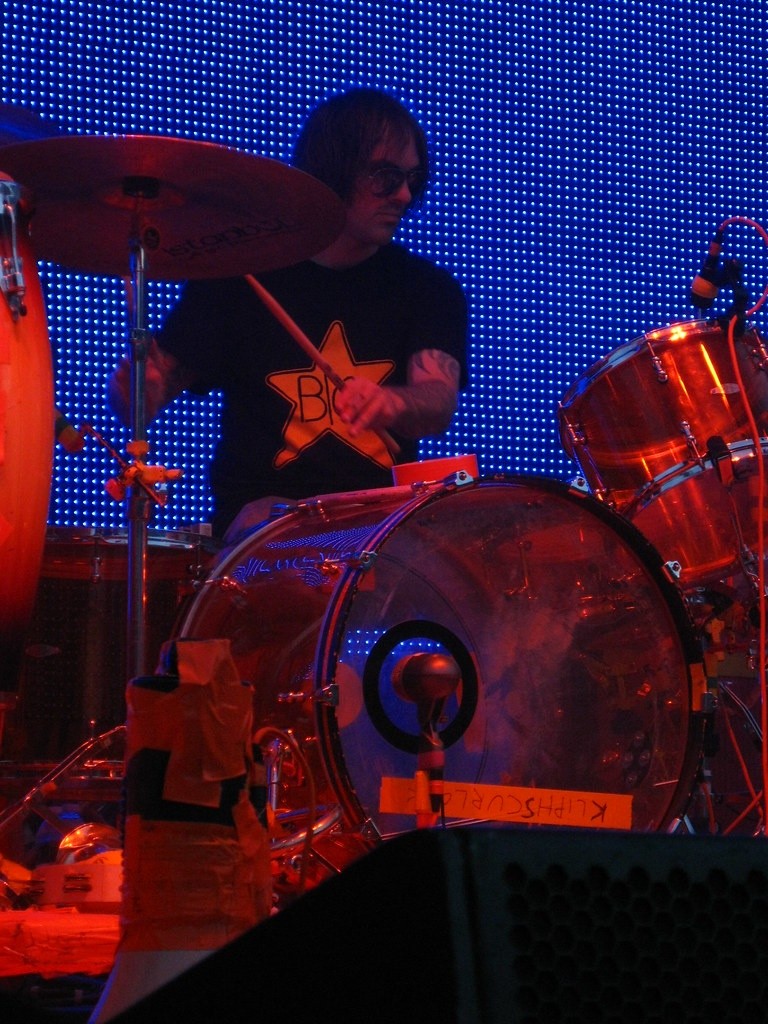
[350,165,427,197]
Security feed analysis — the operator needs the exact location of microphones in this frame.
[691,228,723,308]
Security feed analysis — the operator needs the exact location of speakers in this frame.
[104,820,767,1024]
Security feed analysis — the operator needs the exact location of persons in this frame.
[115,87,471,554]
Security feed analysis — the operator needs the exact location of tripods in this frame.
[0,220,265,891]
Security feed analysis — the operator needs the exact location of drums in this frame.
[6,528,217,805]
[557,317,768,591]
[160,471,710,907]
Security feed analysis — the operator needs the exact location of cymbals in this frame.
[2,135,341,282]
